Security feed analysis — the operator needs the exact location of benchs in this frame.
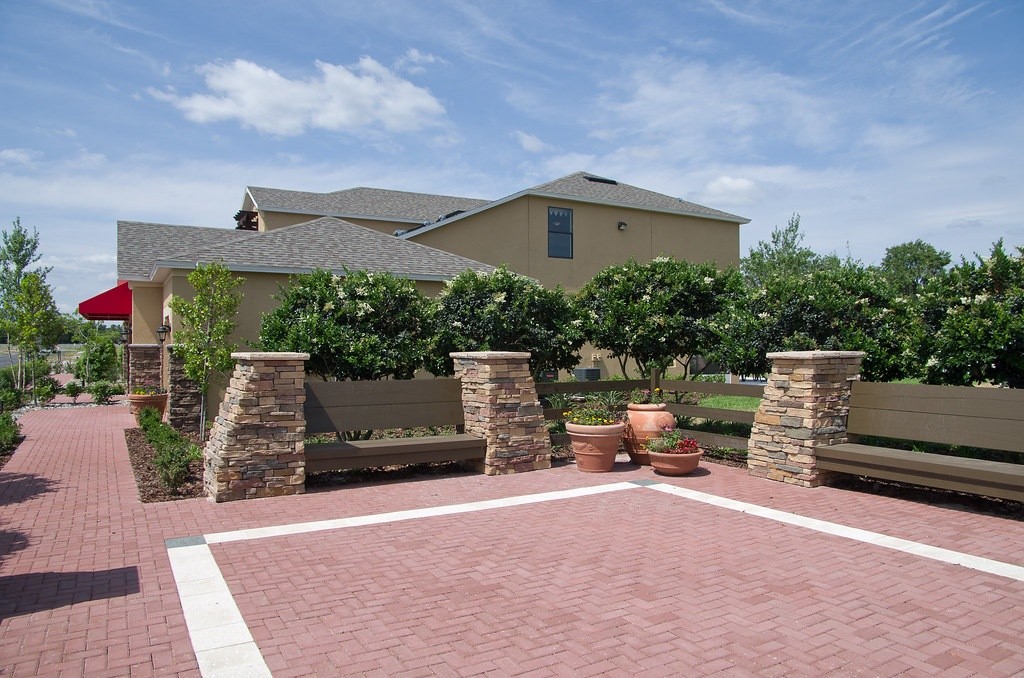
[305,377,487,472]
[815,380,1023,501]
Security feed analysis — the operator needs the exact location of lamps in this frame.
[156,322,171,342]
[618,220,628,231]
[119,327,132,344]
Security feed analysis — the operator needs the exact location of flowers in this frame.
[563,406,622,427]
[639,427,700,455]
[131,385,167,395]
[633,387,665,408]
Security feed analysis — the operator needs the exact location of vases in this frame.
[129,393,169,427]
[566,419,625,472]
[622,402,676,464]
[649,446,703,475]
